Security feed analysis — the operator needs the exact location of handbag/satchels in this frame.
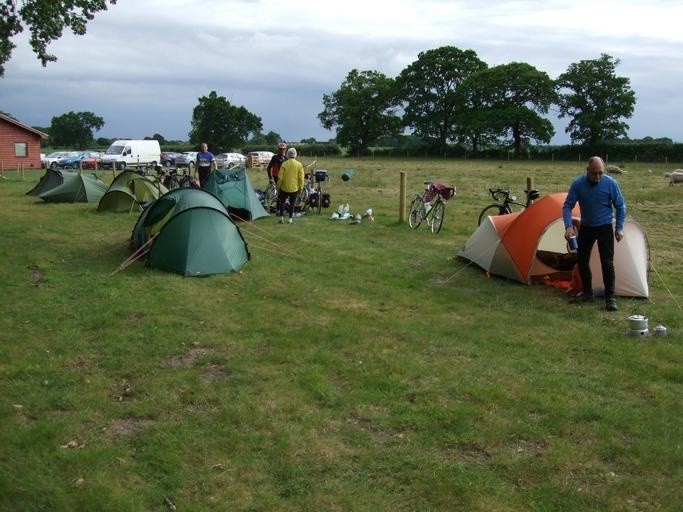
[322,194,330,207]
[309,194,319,207]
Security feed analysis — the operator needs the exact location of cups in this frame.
[344,212,353,219]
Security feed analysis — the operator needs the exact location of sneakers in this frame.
[606,296,618,310]
[569,293,594,304]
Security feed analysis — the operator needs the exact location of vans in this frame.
[100,139,162,170]
[246,151,276,163]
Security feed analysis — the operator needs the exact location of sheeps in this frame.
[605,165,624,176]
[664,169,683,180]
[668,173,683,187]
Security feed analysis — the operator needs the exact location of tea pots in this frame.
[655,325,666,337]
[626,314,649,329]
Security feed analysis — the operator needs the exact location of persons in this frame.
[266,143,288,183]
[194,143,218,188]
[562,155,627,312]
[275,148,305,223]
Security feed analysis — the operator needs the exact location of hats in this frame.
[278,143,287,148]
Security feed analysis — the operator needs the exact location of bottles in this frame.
[568,234,579,250]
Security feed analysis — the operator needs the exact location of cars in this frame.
[81,150,105,169]
[212,152,247,169]
[58,151,83,169]
[40,152,45,160]
[40,152,67,169]
[160,151,179,167]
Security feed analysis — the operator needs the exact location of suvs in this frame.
[174,151,198,168]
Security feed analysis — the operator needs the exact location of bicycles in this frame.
[136,165,152,177]
[264,173,330,215]
[477,187,540,227]
[408,181,456,235]
[155,166,195,189]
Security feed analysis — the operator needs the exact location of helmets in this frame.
[287,148,297,158]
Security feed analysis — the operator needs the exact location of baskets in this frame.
[316,172,325,181]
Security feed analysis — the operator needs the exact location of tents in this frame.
[456,192,649,299]
[97,169,169,213]
[203,167,271,221]
[40,173,110,203]
[130,186,251,278]
[25,169,78,196]
